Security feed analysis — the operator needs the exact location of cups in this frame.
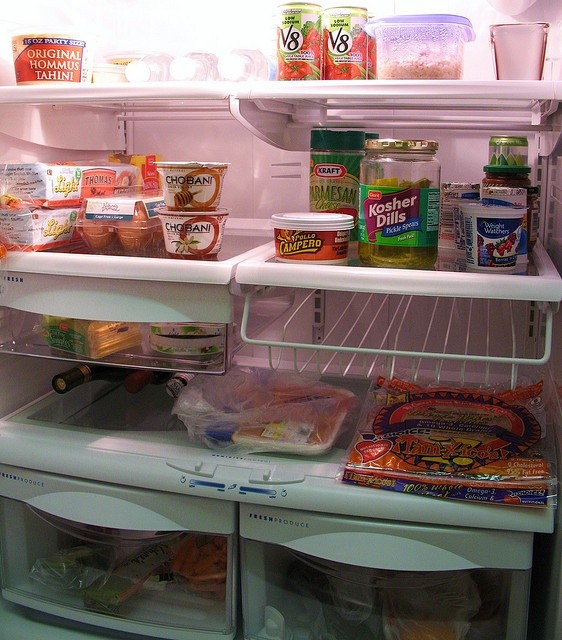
[489,21,550,80]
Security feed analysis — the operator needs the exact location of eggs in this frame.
[79,198,164,252]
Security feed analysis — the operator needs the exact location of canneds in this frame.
[489,135,529,165]
[358,138,442,271]
[367,15,378,80]
[523,187,540,252]
[323,6,367,80]
[276,2,321,81]
[479,165,530,187]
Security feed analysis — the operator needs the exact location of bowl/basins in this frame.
[364,13,476,79]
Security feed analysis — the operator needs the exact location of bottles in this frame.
[217,50,276,80]
[125,53,174,83]
[52,345,142,394]
[165,356,210,400]
[170,53,219,82]
[124,351,175,393]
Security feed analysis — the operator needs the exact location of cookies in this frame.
[33,314,144,360]
